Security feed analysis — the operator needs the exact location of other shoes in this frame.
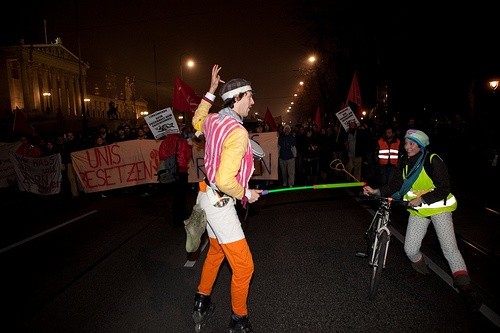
[194,294,215,320]
[463,295,481,321]
[283,183,286,186]
[289,185,293,188]
[230,316,253,333]
[417,276,435,296]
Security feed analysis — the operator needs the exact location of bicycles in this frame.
[362,193,423,301]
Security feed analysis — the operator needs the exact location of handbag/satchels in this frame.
[164,153,177,174]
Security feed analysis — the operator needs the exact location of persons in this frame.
[247,114,469,187]
[159,125,191,228]
[15,121,199,199]
[191,65,263,333]
[362,129,474,293]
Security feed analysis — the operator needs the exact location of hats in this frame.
[219,79,252,100]
[405,130,430,148]
[283,125,291,132]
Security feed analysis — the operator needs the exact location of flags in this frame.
[264,107,279,131]
[347,74,362,112]
[314,108,321,132]
[14,109,36,136]
[172,77,200,114]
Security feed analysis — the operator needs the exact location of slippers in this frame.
[185,206,207,253]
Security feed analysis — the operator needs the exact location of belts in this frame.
[204,178,225,197]
